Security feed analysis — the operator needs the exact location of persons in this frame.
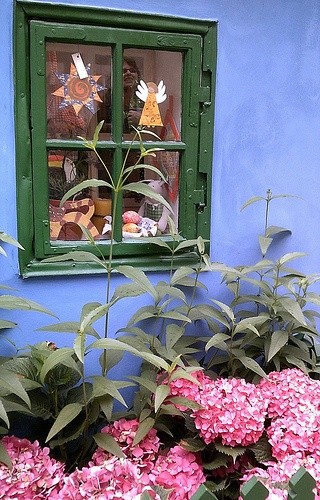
[99,54,147,197]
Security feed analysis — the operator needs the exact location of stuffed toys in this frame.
[136,178,170,234]
